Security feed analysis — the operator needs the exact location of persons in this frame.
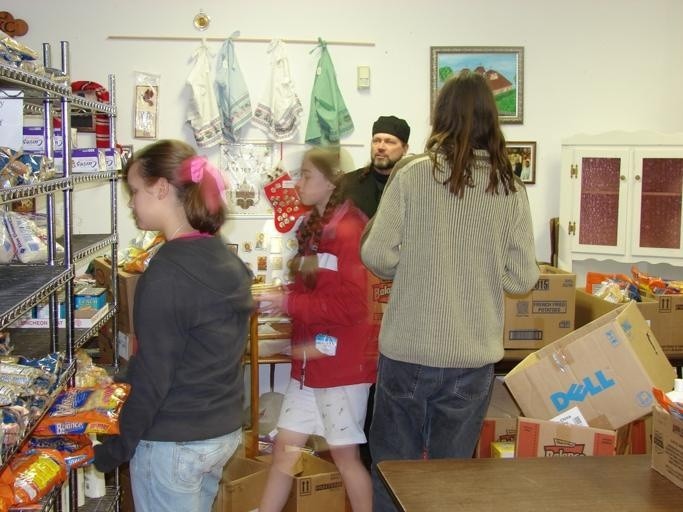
[367,70,543,511]
[339,115,410,216]
[513,150,523,177]
[250,147,385,512]
[93,139,260,512]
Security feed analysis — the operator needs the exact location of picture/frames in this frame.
[429,45,523,125]
[503,141,537,184]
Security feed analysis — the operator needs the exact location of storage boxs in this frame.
[118,451,270,511]
[91,252,143,367]
[254,453,346,511]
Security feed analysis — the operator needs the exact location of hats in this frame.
[371,115,411,144]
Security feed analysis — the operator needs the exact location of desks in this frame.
[377,454,683,512]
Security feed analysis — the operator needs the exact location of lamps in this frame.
[193,12,210,31]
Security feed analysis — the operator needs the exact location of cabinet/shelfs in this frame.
[558,129,683,273]
[242,314,294,457]
